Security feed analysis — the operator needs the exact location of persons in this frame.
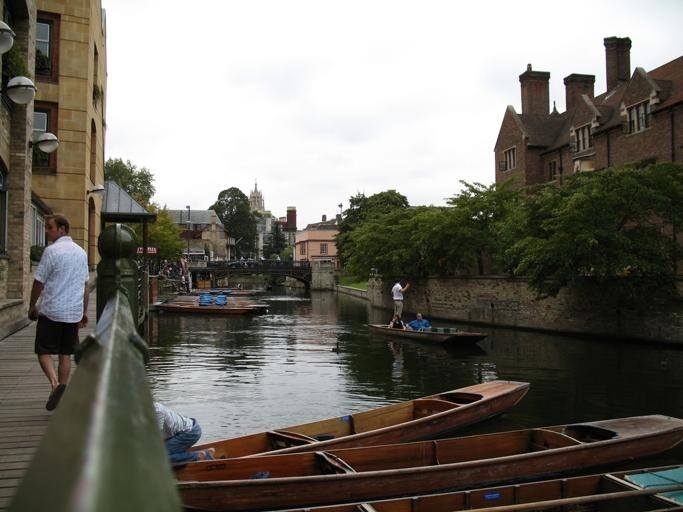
[275,255,280,263]
[148,259,183,291]
[408,342,430,359]
[388,340,403,356]
[259,254,266,263]
[386,342,404,387]
[387,314,407,330]
[26,212,91,412]
[406,312,431,332]
[153,401,217,467]
[390,277,410,320]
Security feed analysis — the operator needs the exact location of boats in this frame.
[367,324,488,344]
[156,297,270,315]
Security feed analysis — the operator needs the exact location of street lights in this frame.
[186,206,191,258]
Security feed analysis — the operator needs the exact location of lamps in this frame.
[88,182,109,204]
[3,71,41,108]
[29,131,60,156]
[0,20,16,55]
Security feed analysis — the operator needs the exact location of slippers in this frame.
[44,383,65,412]
